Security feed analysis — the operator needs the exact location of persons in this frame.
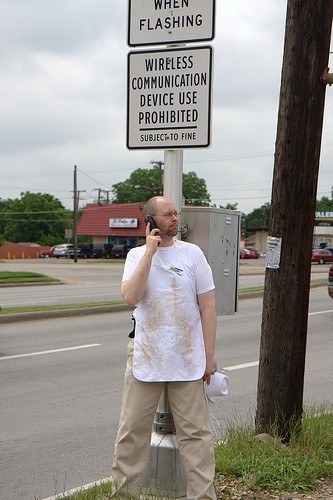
[110,195,217,500]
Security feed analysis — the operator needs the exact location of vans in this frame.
[53,243,81,258]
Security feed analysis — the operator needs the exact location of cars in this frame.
[40,244,59,258]
[239,246,261,260]
[311,241,333,265]
[77,242,128,259]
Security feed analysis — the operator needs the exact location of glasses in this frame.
[150,212,181,219]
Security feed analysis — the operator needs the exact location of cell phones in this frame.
[144,215,158,235]
